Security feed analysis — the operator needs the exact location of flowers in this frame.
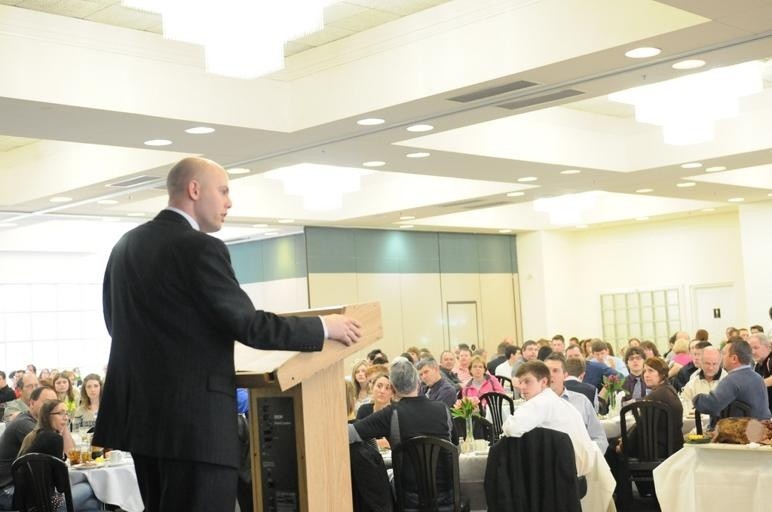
[450,395,480,441]
[64,399,76,427]
[601,373,630,410]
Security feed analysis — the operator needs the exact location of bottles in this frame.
[459,437,465,454]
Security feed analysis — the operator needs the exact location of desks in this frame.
[597,412,709,438]
[377,439,494,512]
[652,441,772,511]
[67,428,146,512]
[486,405,522,424]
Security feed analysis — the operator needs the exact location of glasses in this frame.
[629,355,642,361]
[49,410,66,416]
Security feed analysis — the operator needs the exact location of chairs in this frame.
[479,392,513,445]
[482,428,582,512]
[620,401,674,509]
[236,412,255,509]
[495,376,514,402]
[452,415,493,445]
[350,440,396,511]
[391,436,470,511]
[10,454,73,511]
[696,403,751,435]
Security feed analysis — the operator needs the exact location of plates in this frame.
[73,463,104,469]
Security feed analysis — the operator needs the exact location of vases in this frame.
[464,418,476,452]
[608,392,617,412]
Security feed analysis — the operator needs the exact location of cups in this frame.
[68,448,81,465]
[474,440,487,451]
[110,451,122,463]
[81,447,90,463]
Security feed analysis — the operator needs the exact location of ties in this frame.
[632,378,642,400]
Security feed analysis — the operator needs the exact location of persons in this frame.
[345,335,629,512]
[604,325,771,512]
[89,154,364,512]
[0,362,252,512]
